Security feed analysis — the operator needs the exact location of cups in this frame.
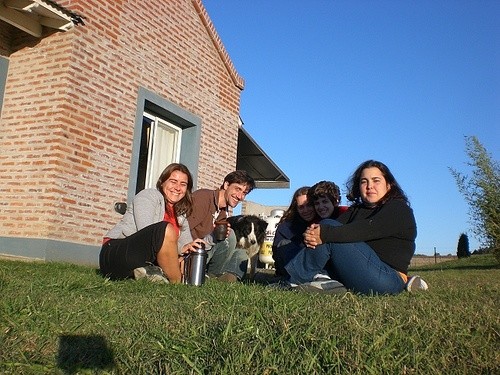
[214,222,228,241]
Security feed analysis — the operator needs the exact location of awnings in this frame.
[235,124,290,190]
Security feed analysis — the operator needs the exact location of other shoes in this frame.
[204,273,237,284]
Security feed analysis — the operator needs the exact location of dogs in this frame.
[227,214,269,279]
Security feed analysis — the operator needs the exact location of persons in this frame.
[187,170,256,283]
[269,160,417,295]
[99,164,206,284]
[272,186,320,280]
[307,181,428,294]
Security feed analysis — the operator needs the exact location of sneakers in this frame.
[265,280,292,291]
[289,274,347,295]
[407,275,429,294]
[134,265,170,285]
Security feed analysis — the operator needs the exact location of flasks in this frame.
[190,242,208,286]
